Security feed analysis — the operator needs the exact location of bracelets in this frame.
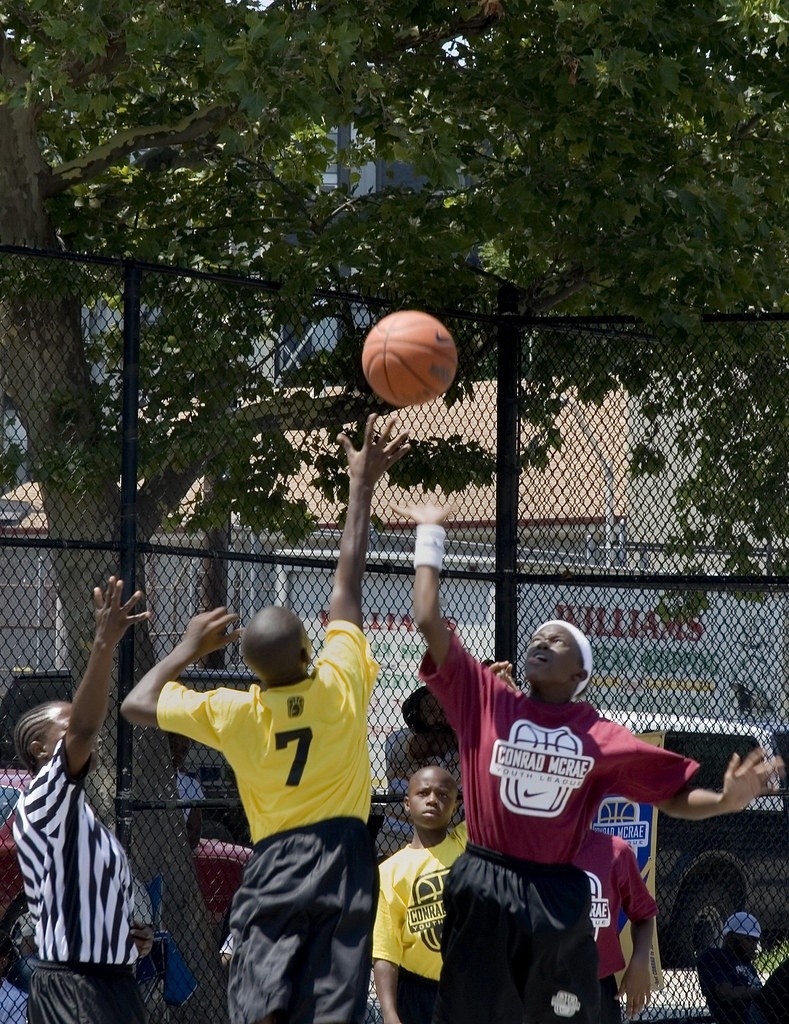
[413,524,446,572]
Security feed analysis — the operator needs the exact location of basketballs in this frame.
[363,308,462,410]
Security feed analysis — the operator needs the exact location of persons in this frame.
[696,911,769,1023]
[573,827,661,1024]
[170,683,464,866]
[11,574,153,1024]
[372,765,470,1024]
[119,411,412,1024]
[392,500,787,1024]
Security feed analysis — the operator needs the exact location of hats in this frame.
[722,912,761,938]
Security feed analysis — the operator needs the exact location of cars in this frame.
[597,709,788,965]
[0,768,255,995]
